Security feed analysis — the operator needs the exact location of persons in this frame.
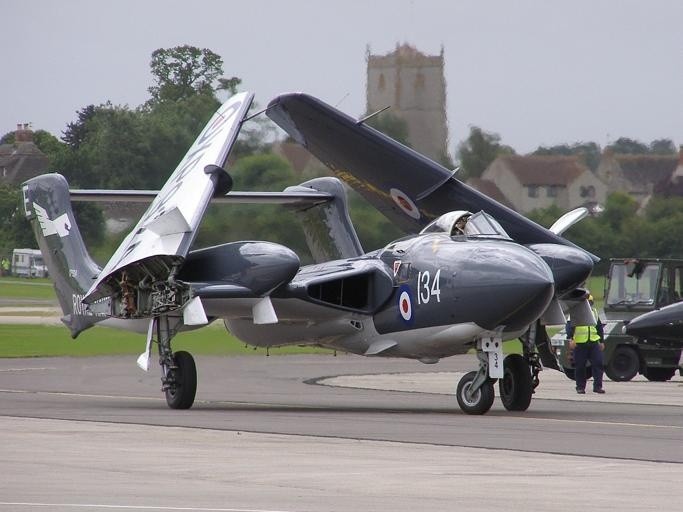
[1,256,11,277]
[566,295,606,393]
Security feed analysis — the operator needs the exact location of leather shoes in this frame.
[592,388,606,394]
[576,387,585,394]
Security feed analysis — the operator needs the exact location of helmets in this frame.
[587,294,593,302]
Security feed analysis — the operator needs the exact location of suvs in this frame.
[550,257,683,381]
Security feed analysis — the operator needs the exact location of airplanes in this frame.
[20,92,601,415]
